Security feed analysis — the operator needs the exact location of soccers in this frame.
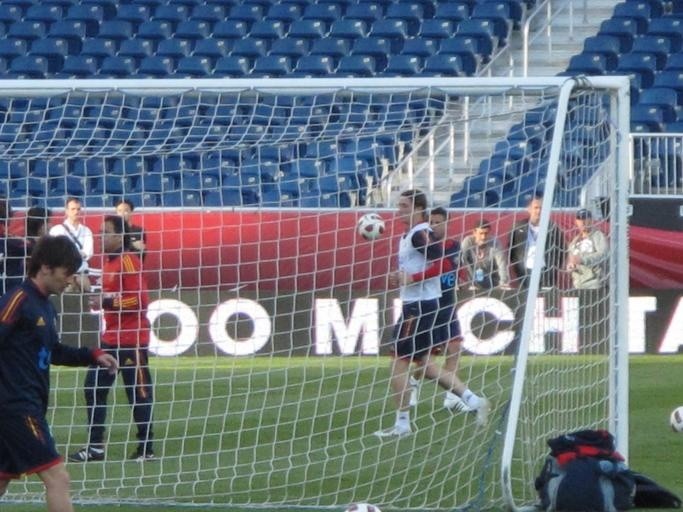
[670,407,683,433]
[358,212,386,240]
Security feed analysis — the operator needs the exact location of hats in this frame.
[574,208,592,220]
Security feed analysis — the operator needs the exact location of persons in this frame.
[457,219,513,294]
[0,237,119,511]
[66,216,153,462]
[374,189,489,439]
[565,212,610,292]
[508,196,563,288]
[0,198,146,298]
[407,207,468,414]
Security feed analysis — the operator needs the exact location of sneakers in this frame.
[67,446,106,463]
[442,396,470,413]
[129,441,154,462]
[475,399,491,432]
[372,423,411,439]
[408,382,420,409]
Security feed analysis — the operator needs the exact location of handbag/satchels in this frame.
[534,431,637,512]
